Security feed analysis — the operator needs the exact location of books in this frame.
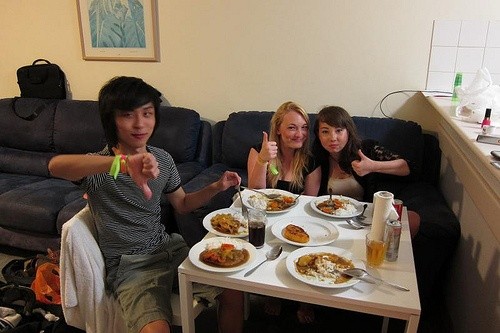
[477,125,500,145]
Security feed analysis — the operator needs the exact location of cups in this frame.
[483,125,495,137]
[366,231,391,271]
[247,209,265,249]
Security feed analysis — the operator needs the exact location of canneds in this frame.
[392,199,403,222]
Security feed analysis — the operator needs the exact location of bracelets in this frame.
[256,155,268,166]
[109,154,128,179]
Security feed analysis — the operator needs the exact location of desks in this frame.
[179,188,421,333]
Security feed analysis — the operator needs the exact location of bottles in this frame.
[452,73,462,101]
[481,108,492,131]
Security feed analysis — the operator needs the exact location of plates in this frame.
[311,195,364,218]
[203,208,268,238]
[188,236,257,273]
[286,246,367,288]
[270,215,339,246]
[240,188,301,214]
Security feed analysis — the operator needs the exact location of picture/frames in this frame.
[76,0,160,62]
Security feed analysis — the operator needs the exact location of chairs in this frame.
[59,204,219,333]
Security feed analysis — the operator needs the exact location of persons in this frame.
[48,76,242,333]
[248,103,321,325]
[312,106,420,239]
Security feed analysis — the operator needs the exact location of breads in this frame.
[283,224,310,243]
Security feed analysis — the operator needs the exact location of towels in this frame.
[59,202,131,333]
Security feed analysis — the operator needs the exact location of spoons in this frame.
[244,245,282,277]
[235,184,281,199]
[343,268,409,292]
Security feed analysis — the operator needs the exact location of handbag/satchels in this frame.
[11,58,67,120]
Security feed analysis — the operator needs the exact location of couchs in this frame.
[0,98,461,305]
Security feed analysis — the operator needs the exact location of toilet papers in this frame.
[370,191,399,241]
